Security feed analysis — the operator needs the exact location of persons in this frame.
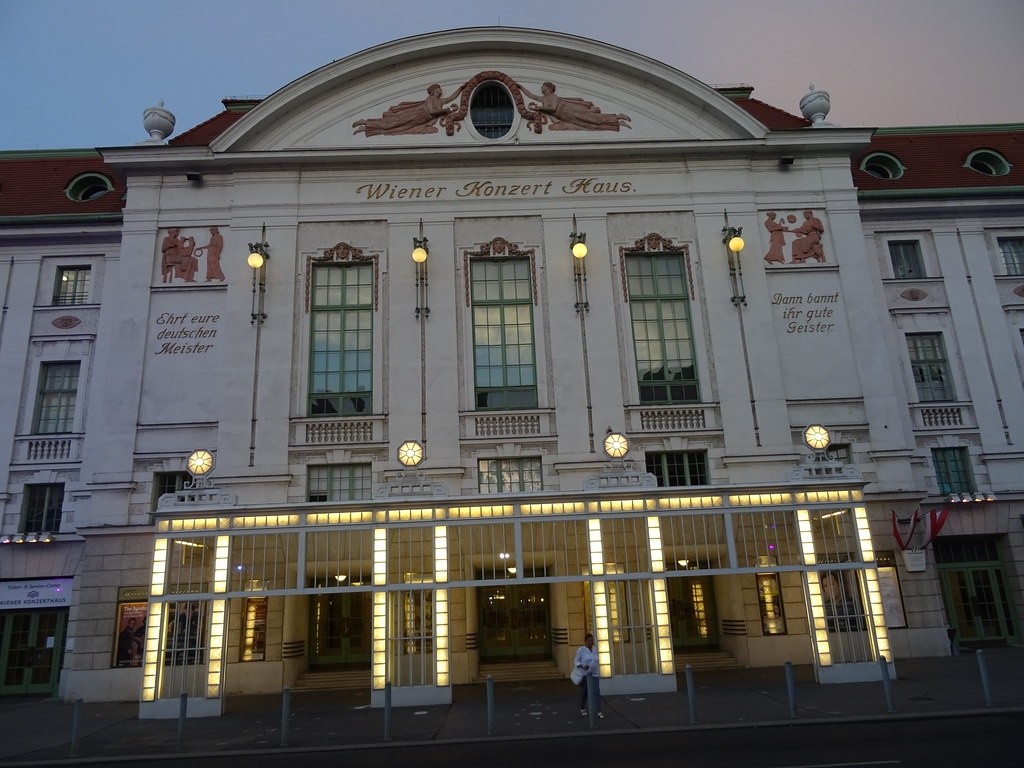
[195,226,225,282]
[119,616,147,667]
[515,76,631,132]
[352,82,471,138]
[822,571,867,632]
[162,227,199,283]
[168,604,206,638]
[764,212,789,264]
[573,633,605,719]
[788,210,824,264]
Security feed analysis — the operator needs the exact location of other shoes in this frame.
[579,709,587,716]
[597,712,604,719]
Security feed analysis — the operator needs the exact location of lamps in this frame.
[412,219,431,319]
[187,172,204,182]
[335,575,347,581]
[25,533,39,543]
[721,208,749,309]
[945,493,961,502]
[247,220,268,326]
[972,492,985,502]
[184,449,217,489]
[603,432,633,473]
[12,533,25,543]
[39,533,56,543]
[778,155,795,164]
[983,492,997,501]
[678,559,690,566]
[569,213,591,314]
[960,493,973,502]
[802,424,835,462]
[396,440,427,480]
[0,535,10,543]
[508,567,517,574]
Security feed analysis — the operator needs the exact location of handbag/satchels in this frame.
[570,666,586,686]
